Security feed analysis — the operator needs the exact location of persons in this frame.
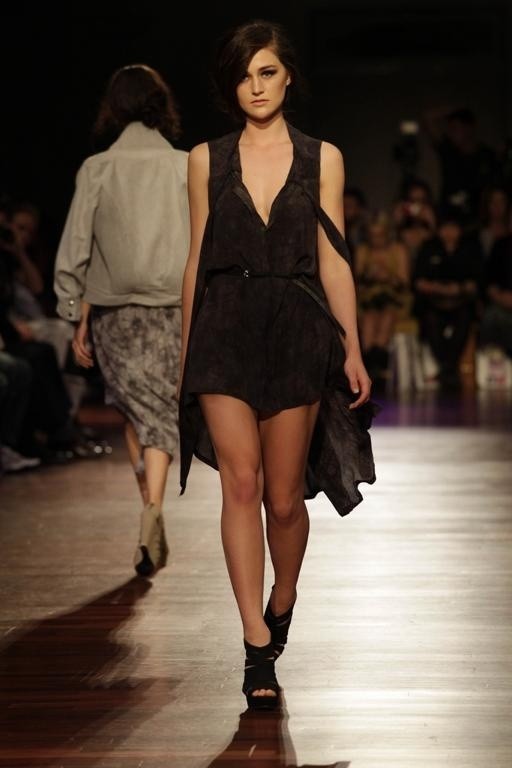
[0,209,123,471]
[54,64,189,577]
[345,185,512,408]
[177,23,371,709]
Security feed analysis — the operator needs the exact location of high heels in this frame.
[134,502,168,576]
[242,585,295,711]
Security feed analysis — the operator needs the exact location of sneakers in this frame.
[0,447,40,471]
